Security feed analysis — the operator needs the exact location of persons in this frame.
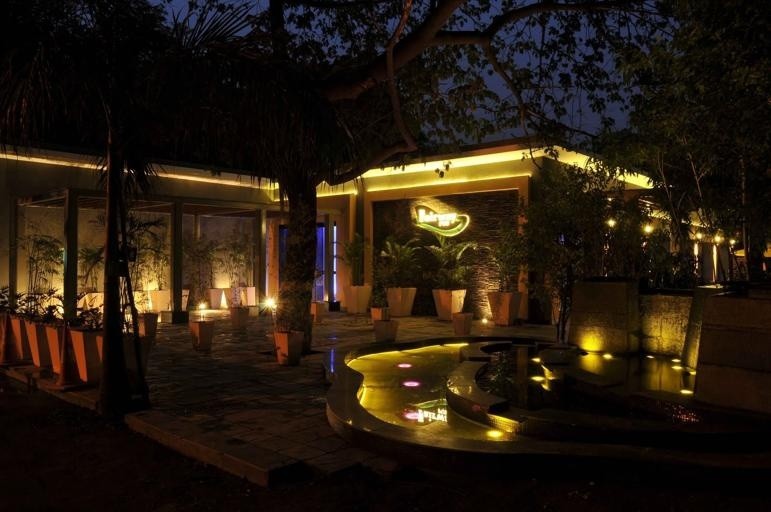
[736,261,746,280]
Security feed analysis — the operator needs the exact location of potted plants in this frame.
[366,260,401,343]
[330,231,374,316]
[2,202,329,394]
[477,203,528,325]
[424,226,494,321]
[447,269,478,335]
[370,221,429,317]
